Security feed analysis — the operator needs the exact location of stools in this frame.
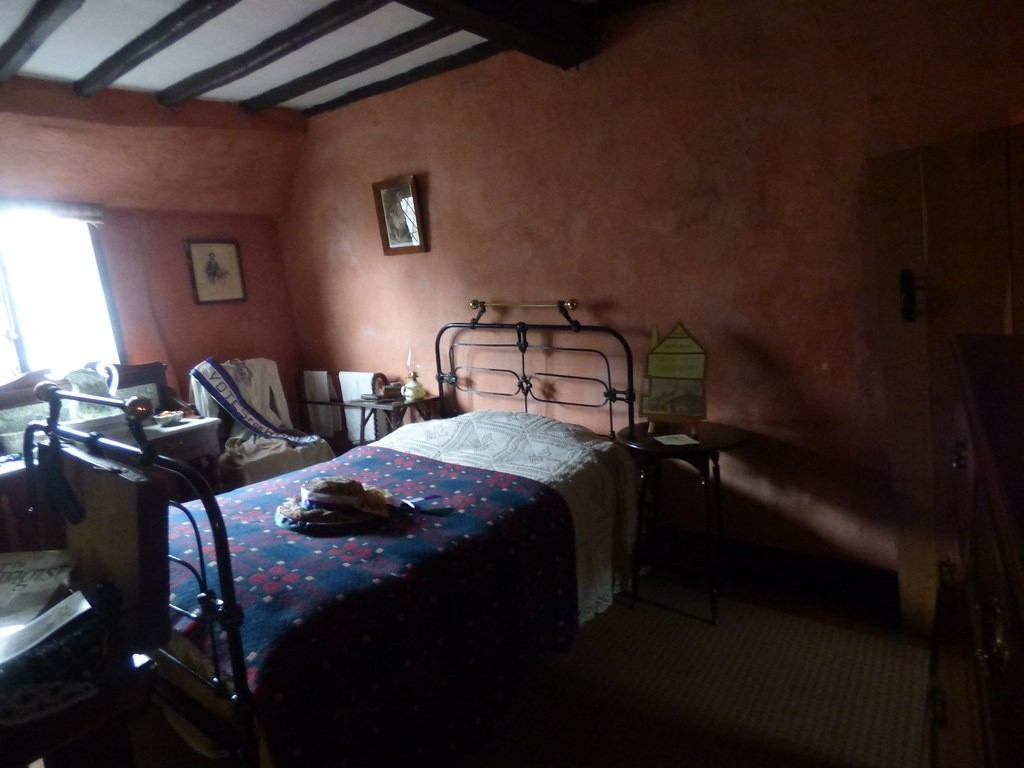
[0,654,133,768]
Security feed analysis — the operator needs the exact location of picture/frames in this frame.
[371,175,429,256]
[185,239,247,306]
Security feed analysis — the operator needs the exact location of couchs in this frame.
[186,358,336,485]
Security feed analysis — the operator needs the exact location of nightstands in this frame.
[344,395,442,443]
[615,423,740,626]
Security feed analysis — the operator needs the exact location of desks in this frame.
[0,413,222,497]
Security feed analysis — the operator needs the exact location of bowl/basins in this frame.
[153,411,184,426]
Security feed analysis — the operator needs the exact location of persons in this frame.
[389,191,406,240]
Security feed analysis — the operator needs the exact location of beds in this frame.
[21,297,637,768]
